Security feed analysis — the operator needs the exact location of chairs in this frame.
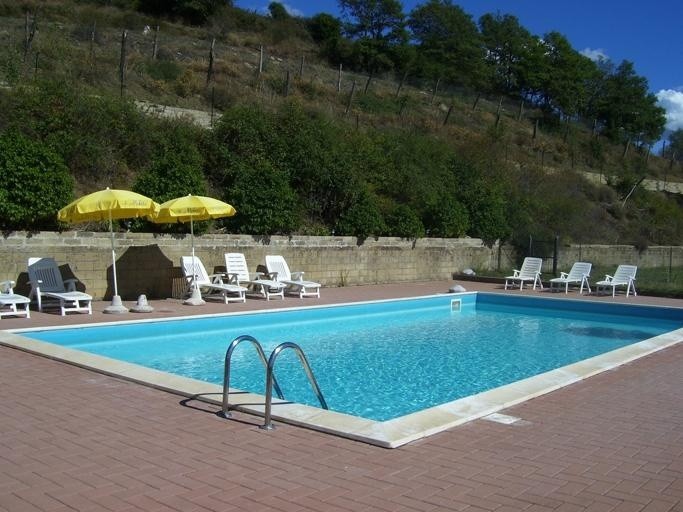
[595,264,638,299]
[548,262,592,295]
[180,252,321,304]
[27,257,93,318]
[0,280,32,321]
[504,257,544,292]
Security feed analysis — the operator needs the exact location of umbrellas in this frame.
[146,193,238,289]
[56,186,161,295]
